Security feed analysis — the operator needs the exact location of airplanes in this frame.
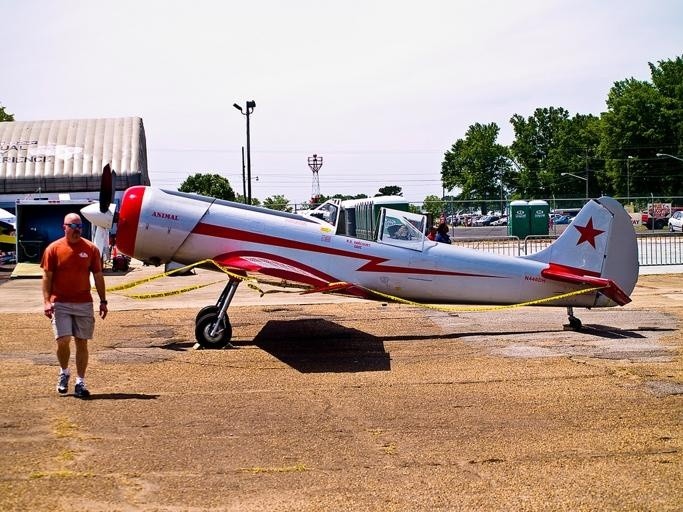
[76,160,644,351]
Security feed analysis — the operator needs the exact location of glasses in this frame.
[65,224,83,229]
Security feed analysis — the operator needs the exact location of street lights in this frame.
[655,152,683,162]
[243,176,260,203]
[230,98,258,206]
[560,172,588,198]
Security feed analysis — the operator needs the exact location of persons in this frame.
[440,213,447,224]
[425,226,437,241]
[38,213,108,398]
[435,222,451,243]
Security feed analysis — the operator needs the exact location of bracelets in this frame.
[100,300,107,304]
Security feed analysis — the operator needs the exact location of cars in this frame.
[435,206,683,234]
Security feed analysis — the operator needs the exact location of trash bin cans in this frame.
[509,200,549,240]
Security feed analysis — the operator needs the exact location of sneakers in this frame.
[74,384,89,397]
[57,373,69,394]
